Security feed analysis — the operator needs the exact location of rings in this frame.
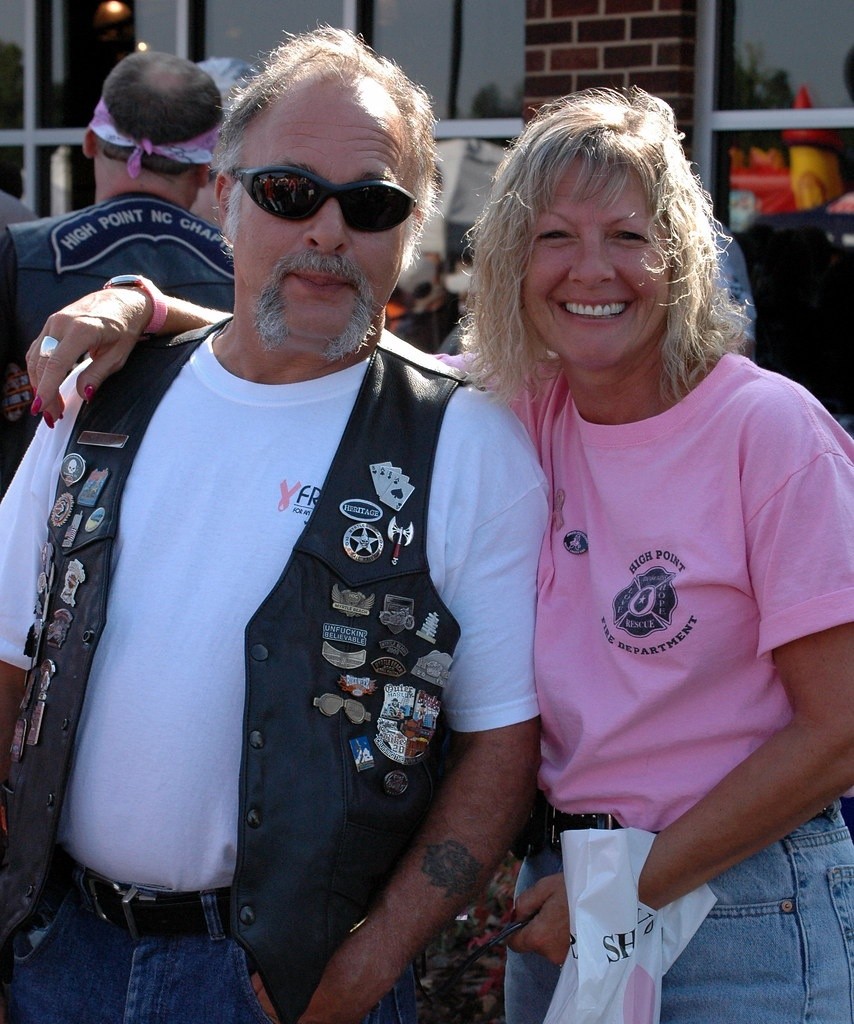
[39,334,59,358]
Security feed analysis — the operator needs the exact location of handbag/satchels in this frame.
[542,827,718,1024]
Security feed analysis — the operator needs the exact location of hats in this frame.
[444,267,475,295]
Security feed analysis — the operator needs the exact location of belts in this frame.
[47,854,232,941]
[531,794,828,851]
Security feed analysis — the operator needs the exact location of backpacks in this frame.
[733,198,854,415]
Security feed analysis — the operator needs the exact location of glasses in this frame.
[233,165,418,232]
[410,851,529,1024]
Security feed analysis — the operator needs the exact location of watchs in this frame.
[103,274,168,341]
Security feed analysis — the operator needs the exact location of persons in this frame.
[0,21,854,1024]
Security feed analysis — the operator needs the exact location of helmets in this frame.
[197,58,260,132]
